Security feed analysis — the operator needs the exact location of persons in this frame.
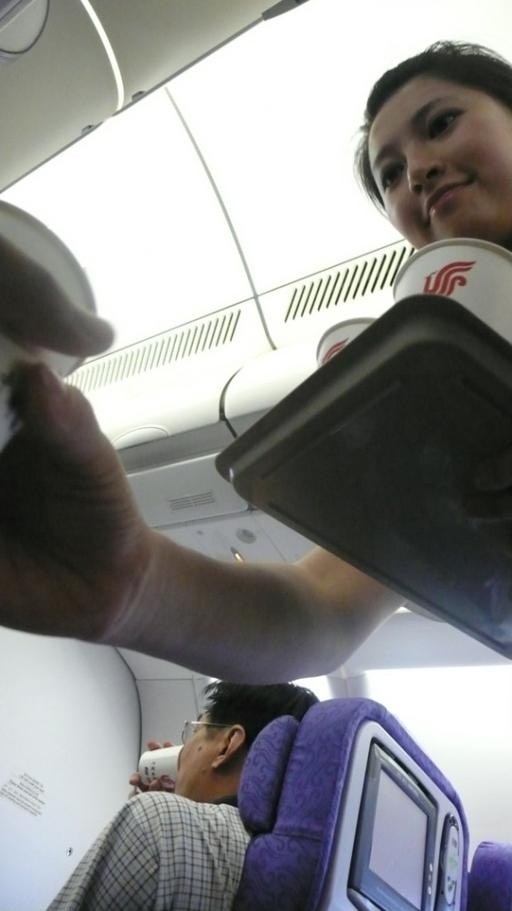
[2,235,114,355]
[46,678,321,910]
[1,39,509,688]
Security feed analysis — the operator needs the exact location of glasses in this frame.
[182,721,232,746]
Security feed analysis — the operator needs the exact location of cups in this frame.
[317,317,377,369]
[0,199,96,455]
[393,237,512,344]
[138,744,185,786]
